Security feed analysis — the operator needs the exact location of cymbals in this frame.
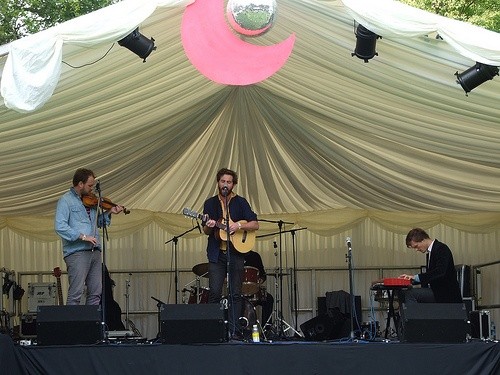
[192,263,208,277]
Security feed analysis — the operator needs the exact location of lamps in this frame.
[454,61,500,97]
[351,19,382,64]
[118,26,157,64]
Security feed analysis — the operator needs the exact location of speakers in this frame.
[299,312,339,342]
[159,303,226,344]
[36,305,103,346]
[316,295,361,339]
[469,311,491,340]
[396,303,468,344]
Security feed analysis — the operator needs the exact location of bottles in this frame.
[252,325,259,342]
[491,322,496,340]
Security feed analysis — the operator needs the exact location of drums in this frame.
[242,266,259,295]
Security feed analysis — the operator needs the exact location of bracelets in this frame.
[82,234,86,240]
[236,221,242,230]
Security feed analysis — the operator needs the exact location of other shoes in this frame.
[227,333,249,342]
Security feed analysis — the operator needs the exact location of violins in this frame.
[81,193,130,216]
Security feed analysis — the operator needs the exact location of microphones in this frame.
[223,182,228,194]
[345,236,352,251]
[92,180,100,194]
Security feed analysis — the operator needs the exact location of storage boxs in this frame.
[26,282,59,314]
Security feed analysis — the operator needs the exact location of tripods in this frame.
[241,219,308,342]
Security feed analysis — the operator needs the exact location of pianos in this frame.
[369,280,413,341]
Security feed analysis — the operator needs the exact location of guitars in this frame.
[182,207,256,253]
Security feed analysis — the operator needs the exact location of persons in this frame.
[200,168,272,340]
[55,168,122,306]
[398,226,460,302]
